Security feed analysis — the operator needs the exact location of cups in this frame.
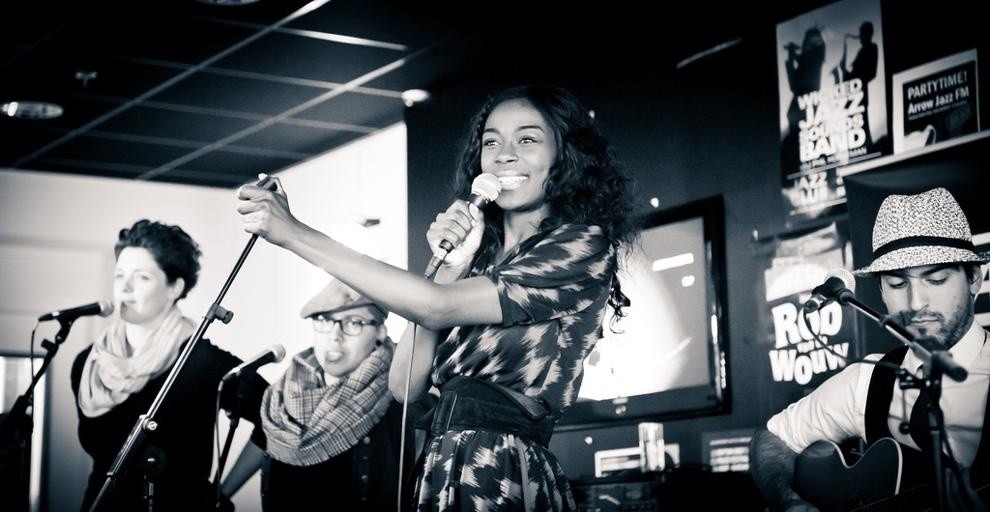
[638,422,665,473]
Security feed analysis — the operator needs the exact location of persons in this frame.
[237,88,632,512]
[221,279,437,512]
[71,220,271,512]
[841,22,878,145]
[786,29,825,138]
[748,188,990,512]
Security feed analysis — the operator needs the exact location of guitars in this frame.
[792,437,990,508]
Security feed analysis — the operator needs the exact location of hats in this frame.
[300,279,388,320]
[851,188,989,277]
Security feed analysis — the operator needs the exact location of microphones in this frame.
[248,170,278,198]
[38,298,116,323]
[803,267,858,316]
[424,172,502,279]
[221,343,288,379]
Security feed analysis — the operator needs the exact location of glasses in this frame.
[310,314,375,337]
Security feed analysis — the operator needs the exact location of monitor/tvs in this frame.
[557,192,732,424]
